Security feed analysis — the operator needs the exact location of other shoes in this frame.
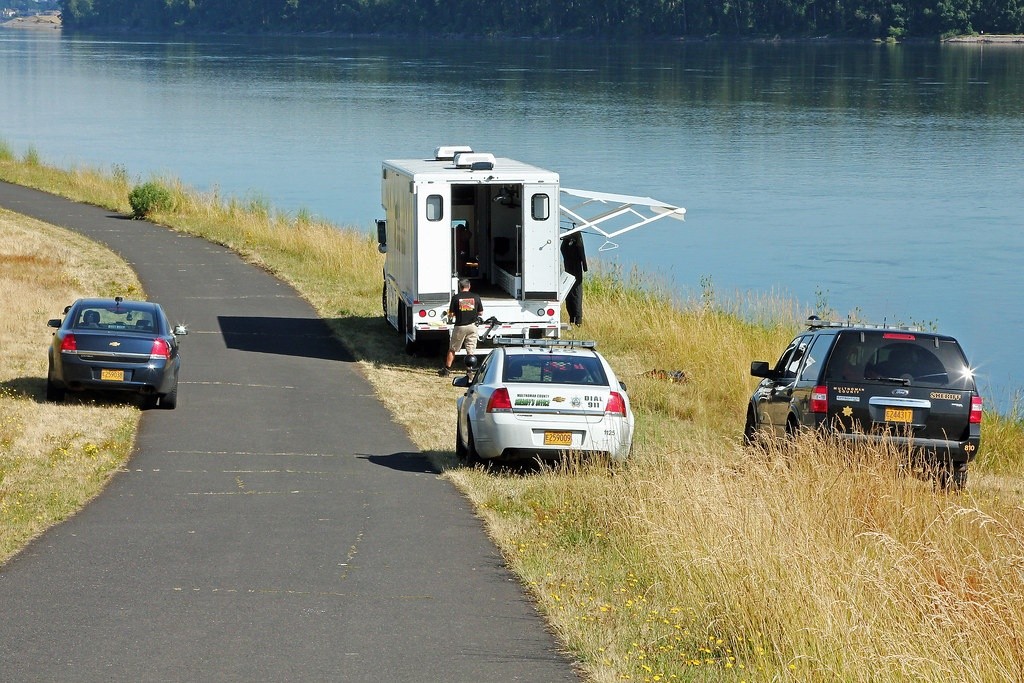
[439,368,450,377]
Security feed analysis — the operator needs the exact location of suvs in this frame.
[743,316,986,493]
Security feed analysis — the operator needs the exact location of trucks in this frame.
[372,146,688,361]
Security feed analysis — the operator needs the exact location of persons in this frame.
[438,279,484,376]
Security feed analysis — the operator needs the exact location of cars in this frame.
[453,336,635,470]
[46,295,188,412]
